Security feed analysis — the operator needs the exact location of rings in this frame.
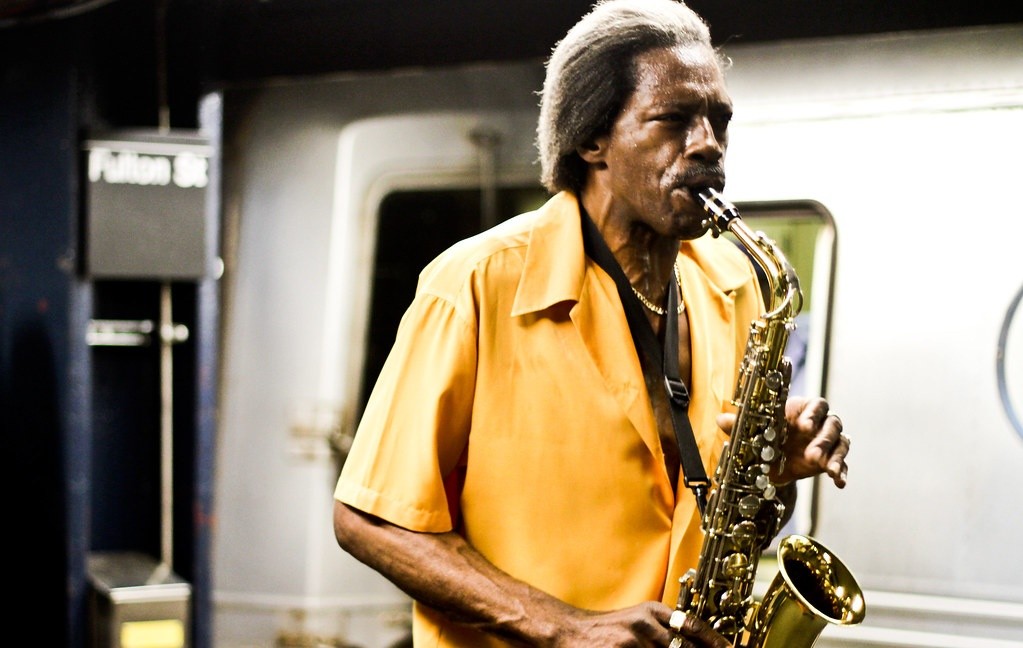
[667,610,689,632]
[668,634,684,648]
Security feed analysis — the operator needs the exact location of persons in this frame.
[334,0,851,648]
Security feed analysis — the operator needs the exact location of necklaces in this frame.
[597,240,691,318]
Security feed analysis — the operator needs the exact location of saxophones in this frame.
[676,183,867,648]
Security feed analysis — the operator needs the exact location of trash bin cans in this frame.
[77,551,192,648]
